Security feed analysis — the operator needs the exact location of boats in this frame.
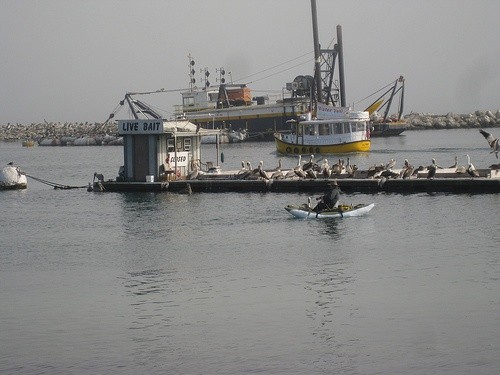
[283,203,375,219]
[171,0,412,145]
[273,100,371,156]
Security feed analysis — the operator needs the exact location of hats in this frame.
[327,181,339,186]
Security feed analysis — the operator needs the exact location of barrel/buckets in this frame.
[146,175,155,182]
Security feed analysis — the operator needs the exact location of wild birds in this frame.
[322,168,330,180]
[294,154,308,181]
[285,167,295,180]
[448,153,458,168]
[271,168,283,180]
[241,160,245,168]
[466,154,480,178]
[186,169,199,180]
[6,160,16,166]
[93,171,104,181]
[321,158,329,170]
[303,154,321,179]
[274,159,281,170]
[259,160,263,166]
[411,165,424,177]
[247,161,252,169]
[365,157,415,185]
[479,128,500,160]
[428,158,438,175]
[234,169,252,180]
[259,169,270,181]
[331,157,359,179]
[252,164,262,175]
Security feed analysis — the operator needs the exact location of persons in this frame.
[311,180,341,213]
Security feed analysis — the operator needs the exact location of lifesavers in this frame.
[286,147,292,153]
[316,148,319,153]
[294,148,299,153]
[309,148,313,153]
[302,148,306,153]
